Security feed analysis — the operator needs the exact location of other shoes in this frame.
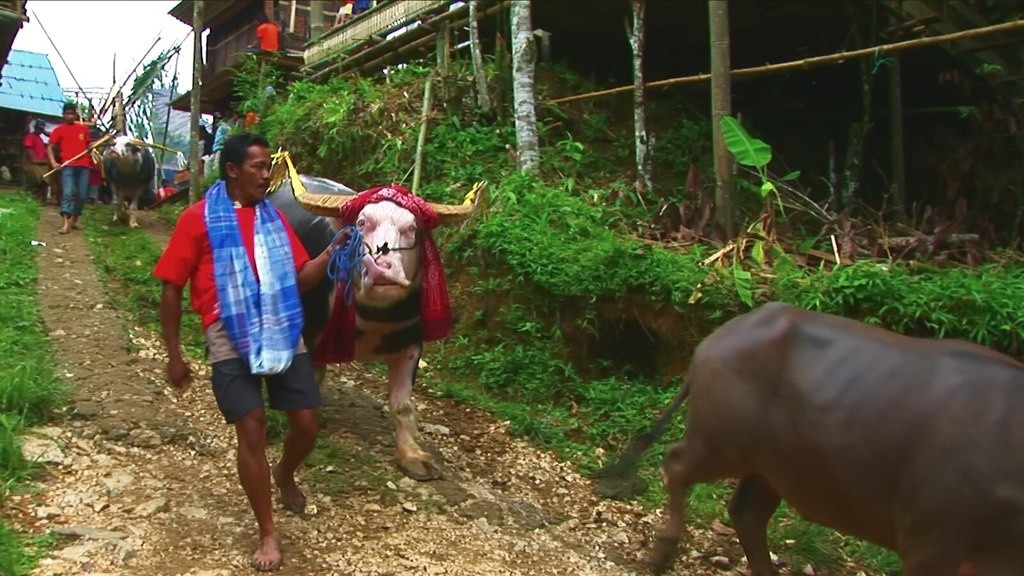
[59,228,72,234]
[70,223,85,230]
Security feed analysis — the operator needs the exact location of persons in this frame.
[244,11,282,57]
[157,177,176,202]
[23,120,48,202]
[201,110,260,161]
[46,102,96,235]
[151,135,364,570]
[88,159,100,205]
[338,8,364,23]
[0,160,12,186]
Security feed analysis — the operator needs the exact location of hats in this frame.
[35,119,46,129]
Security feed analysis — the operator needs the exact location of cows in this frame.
[263,152,488,481]
[587,301,1024,576]
[100,135,155,228]
